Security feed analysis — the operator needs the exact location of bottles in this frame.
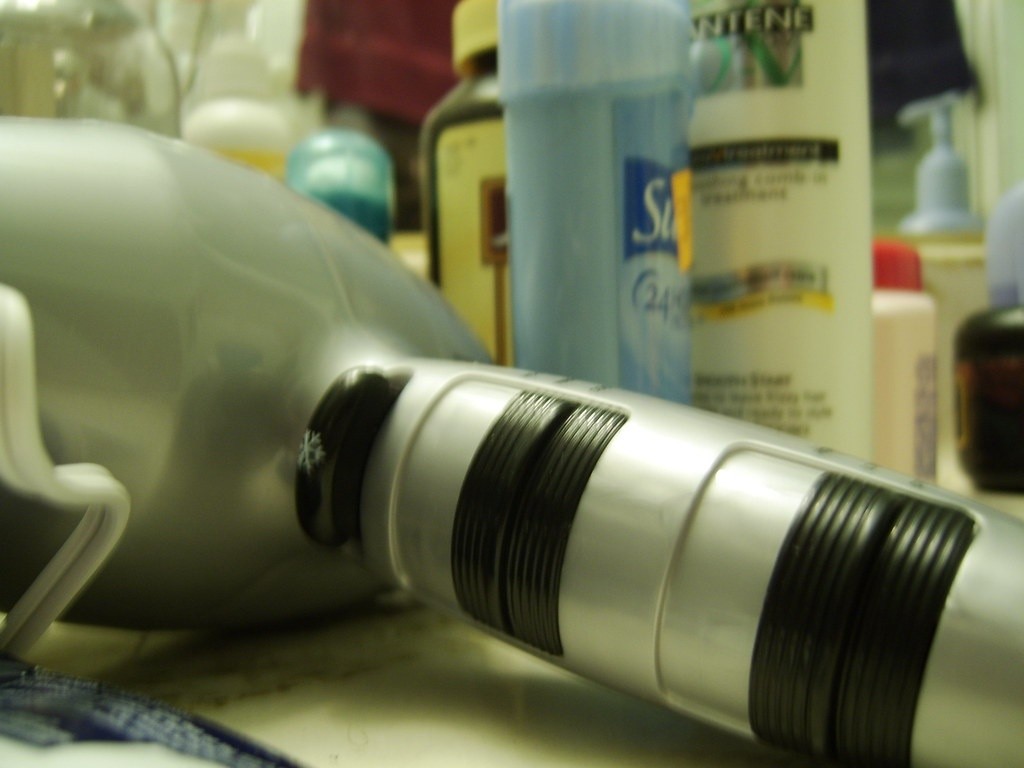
[415,0,510,364]
